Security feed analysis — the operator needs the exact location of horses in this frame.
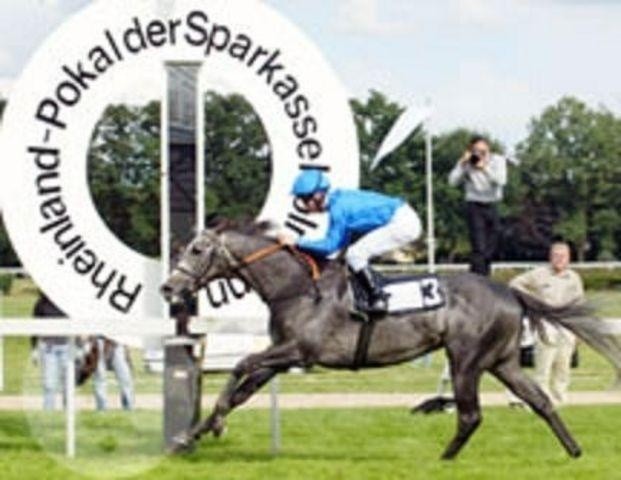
[160,215,620,462]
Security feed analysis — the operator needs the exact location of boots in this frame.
[358,267,387,312]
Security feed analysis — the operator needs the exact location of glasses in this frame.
[301,193,313,203]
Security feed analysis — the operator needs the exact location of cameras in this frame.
[470,148,481,164]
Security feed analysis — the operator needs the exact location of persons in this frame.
[448,136,506,277]
[508,243,585,403]
[86,334,134,410]
[31,296,87,410]
[277,170,423,316]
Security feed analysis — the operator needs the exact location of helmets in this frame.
[292,170,330,196]
